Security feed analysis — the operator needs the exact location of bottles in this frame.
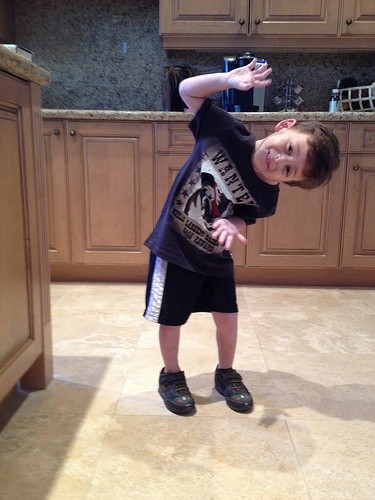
[330,89,339,112]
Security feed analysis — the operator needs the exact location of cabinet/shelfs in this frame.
[159,0,375,53]
[0,70,375,403]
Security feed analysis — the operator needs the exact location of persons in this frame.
[142,57,341,417]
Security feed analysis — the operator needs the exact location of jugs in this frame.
[163,66,194,112]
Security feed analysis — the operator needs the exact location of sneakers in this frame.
[214,370,254,411]
[158,367,195,414]
